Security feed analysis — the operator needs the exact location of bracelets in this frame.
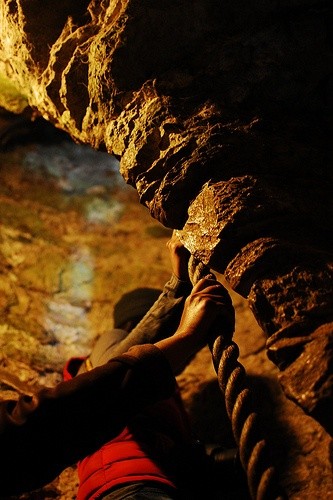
[164,274,187,293]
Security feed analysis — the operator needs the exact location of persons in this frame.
[58,240,207,500]
[0,265,237,500]
